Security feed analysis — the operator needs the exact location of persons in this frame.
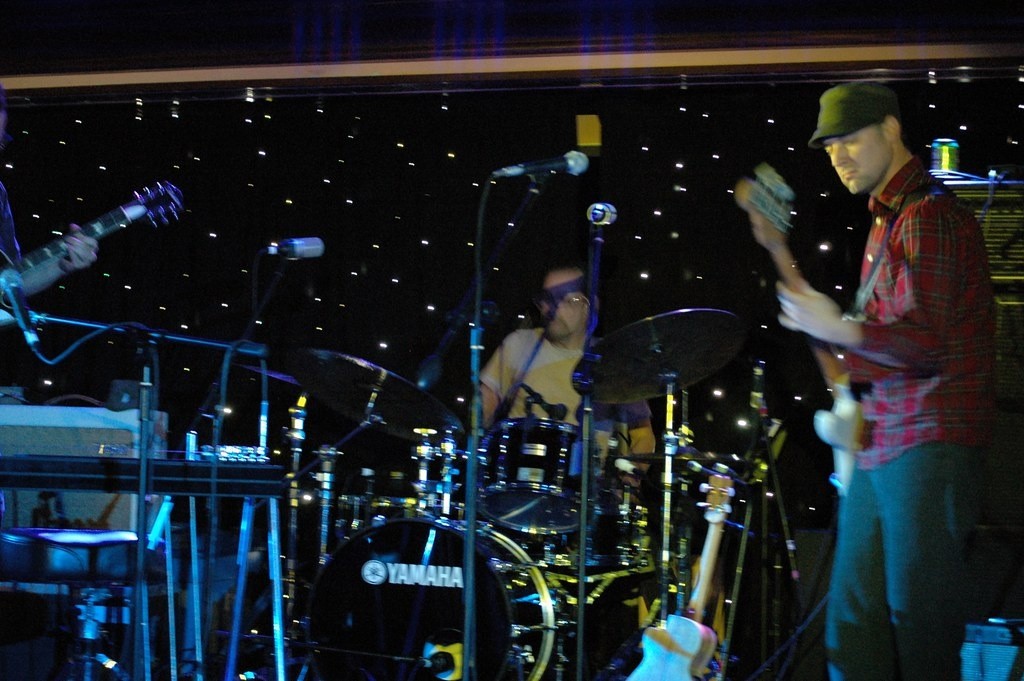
[0,85,98,305]
[467,264,656,486]
[776,81,996,681]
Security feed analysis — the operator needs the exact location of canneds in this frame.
[930,139,961,170]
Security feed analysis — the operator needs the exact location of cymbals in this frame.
[571,307,747,405]
[634,446,728,462]
[280,345,467,444]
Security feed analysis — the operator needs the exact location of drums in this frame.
[646,451,760,565]
[480,418,597,535]
[304,516,559,681]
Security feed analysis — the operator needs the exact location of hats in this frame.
[808,81,896,149]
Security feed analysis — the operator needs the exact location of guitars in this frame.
[0,178,188,342]
[627,474,738,681]
[733,156,872,494]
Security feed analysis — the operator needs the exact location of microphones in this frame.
[266,238,325,258]
[749,363,765,429]
[493,151,589,178]
[423,652,454,678]
[598,436,619,508]
[539,277,588,302]
[522,382,564,419]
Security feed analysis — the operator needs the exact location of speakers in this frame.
[967,288,1024,526]
[960,624,1024,681]
[788,523,1024,681]
